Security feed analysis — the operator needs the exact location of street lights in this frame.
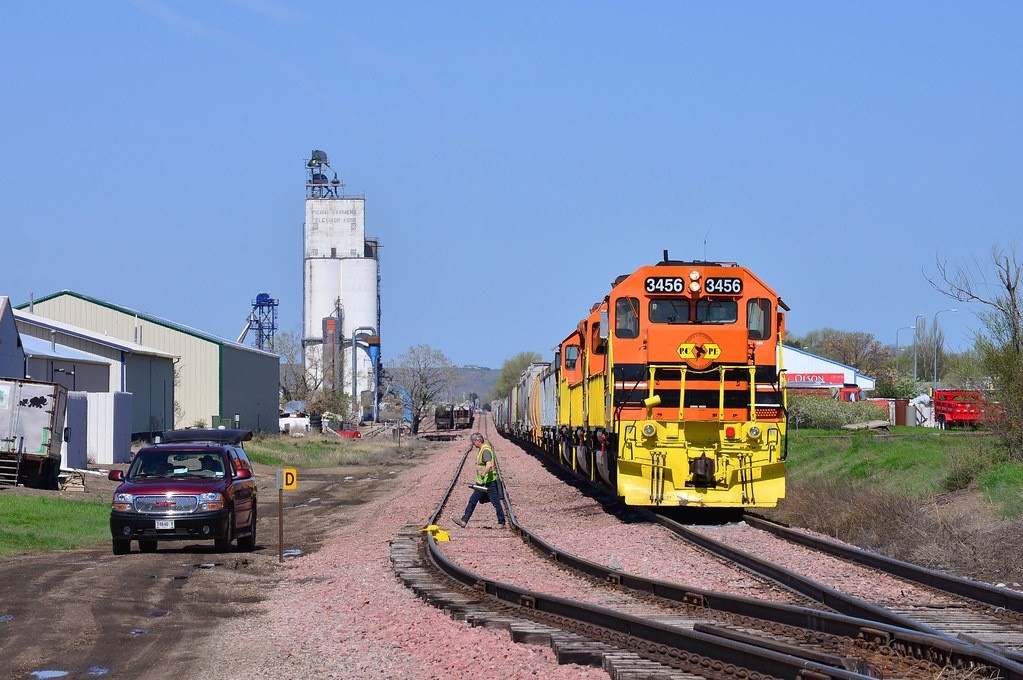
[934,309,959,391]
[897,326,917,371]
[914,314,923,382]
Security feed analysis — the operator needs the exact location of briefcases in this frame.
[479,481,504,504]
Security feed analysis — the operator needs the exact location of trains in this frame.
[490,248,792,509]
[435,399,477,430]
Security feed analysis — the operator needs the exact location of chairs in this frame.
[201,459,222,472]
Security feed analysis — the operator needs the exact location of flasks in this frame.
[468,483,488,493]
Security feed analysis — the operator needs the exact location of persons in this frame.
[200,455,213,470]
[452,432,506,530]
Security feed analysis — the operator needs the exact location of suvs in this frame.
[107,429,259,554]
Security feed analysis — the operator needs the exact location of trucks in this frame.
[786,384,890,418]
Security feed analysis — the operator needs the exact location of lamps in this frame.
[66,371,75,376]
[54,368,65,372]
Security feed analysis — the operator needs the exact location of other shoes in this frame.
[495,524,504,529]
[452,518,466,528]
